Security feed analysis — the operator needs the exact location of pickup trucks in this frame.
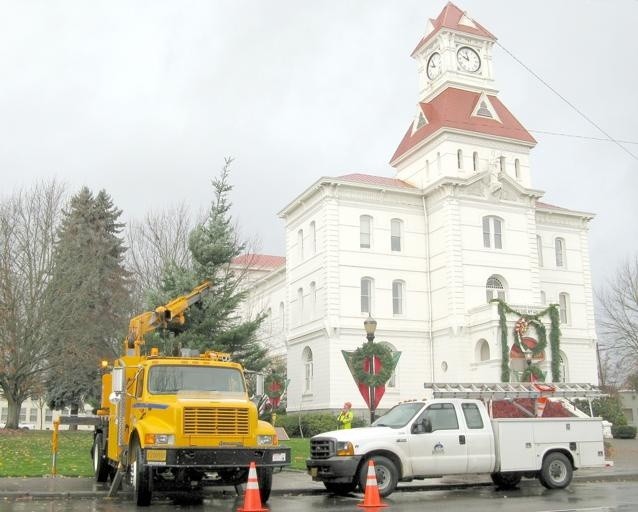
[301,379,614,497]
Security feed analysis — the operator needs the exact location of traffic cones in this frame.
[356,459,386,507]
[237,463,267,512]
[532,385,554,418]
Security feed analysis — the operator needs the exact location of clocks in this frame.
[455,45,484,75]
[424,49,445,82]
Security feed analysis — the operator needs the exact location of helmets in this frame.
[344,402,353,408]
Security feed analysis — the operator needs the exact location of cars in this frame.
[0,420,36,430]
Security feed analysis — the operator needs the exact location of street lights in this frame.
[362,311,378,423]
[524,347,533,377]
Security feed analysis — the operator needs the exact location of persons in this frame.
[259,402,274,428]
[335,401,355,430]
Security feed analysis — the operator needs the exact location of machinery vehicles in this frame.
[49,278,294,504]
